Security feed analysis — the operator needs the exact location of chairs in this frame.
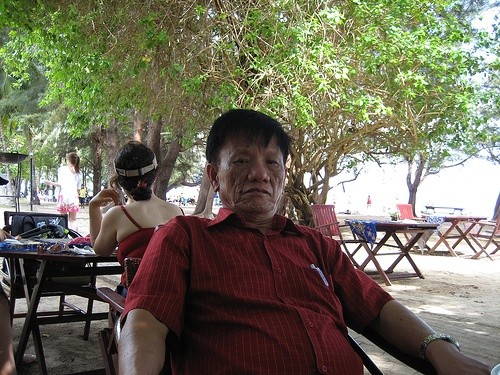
[4,211,98,341]
[395,203,432,252]
[96,256,143,374]
[308,205,360,270]
[468,213,500,261]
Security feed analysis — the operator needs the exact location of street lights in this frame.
[29,151,34,211]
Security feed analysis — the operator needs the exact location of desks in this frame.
[0,243,121,375]
[421,215,487,257]
[344,220,440,279]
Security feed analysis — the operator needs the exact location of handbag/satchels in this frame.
[20,215,69,239]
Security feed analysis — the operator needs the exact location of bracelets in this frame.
[419,332,460,363]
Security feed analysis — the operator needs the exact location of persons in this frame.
[88,139,185,294]
[78,182,373,209]
[0,176,17,375]
[115,108,491,374]
[41,152,84,229]
